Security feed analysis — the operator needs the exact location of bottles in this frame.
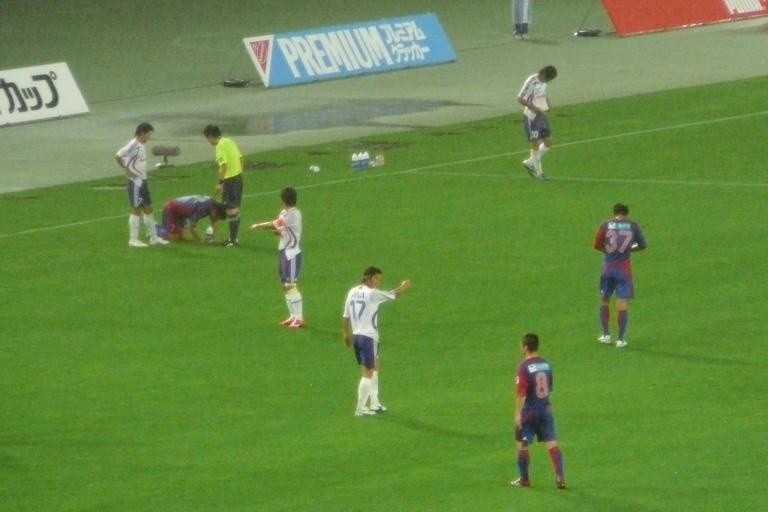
[308,165,321,173]
[351,150,386,172]
[206,224,214,243]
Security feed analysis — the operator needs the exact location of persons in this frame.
[517,66,557,180]
[512,0,533,40]
[510,334,566,489]
[343,267,411,417]
[594,204,647,348]
[115,122,169,248]
[249,187,305,328]
[204,126,241,246]
[155,195,227,244]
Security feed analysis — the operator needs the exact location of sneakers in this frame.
[617,339,627,349]
[521,158,545,179]
[510,478,531,489]
[600,334,611,344]
[129,237,240,248]
[281,318,305,328]
[354,404,389,416]
[556,480,566,490]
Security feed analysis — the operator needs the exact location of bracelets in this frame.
[218,180,224,184]
